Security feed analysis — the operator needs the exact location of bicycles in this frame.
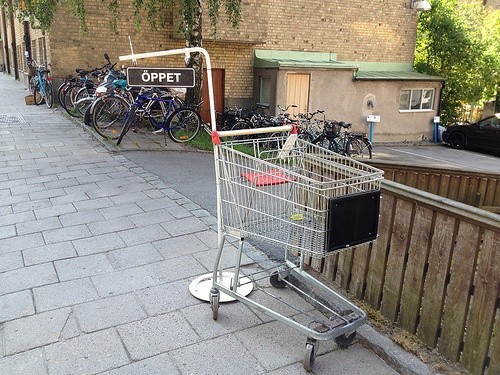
[25,50,54,109]
[215,102,373,164]
[58,53,204,146]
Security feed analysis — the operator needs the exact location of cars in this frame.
[442,112,500,158]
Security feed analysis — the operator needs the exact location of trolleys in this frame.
[209,124,385,371]
